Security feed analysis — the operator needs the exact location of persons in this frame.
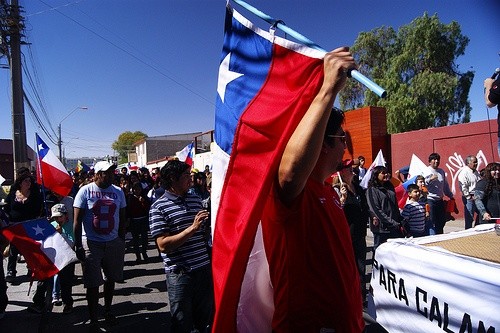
[458,156,480,230]
[72,161,127,329]
[113,167,160,263]
[474,162,500,226]
[262,47,363,333]
[395,166,409,183]
[189,165,212,206]
[149,160,215,333]
[400,184,425,238]
[366,166,404,248]
[415,175,428,207]
[0,167,95,318]
[352,156,367,181]
[424,152,453,235]
[483,75,500,158]
[333,159,378,306]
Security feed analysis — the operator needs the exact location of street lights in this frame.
[58,106,89,162]
[62,137,79,165]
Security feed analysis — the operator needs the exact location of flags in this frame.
[395,176,418,210]
[210,4,328,333]
[34,134,74,197]
[2,218,78,281]
[359,150,385,189]
[178,143,193,166]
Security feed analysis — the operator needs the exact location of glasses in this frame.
[326,130,346,144]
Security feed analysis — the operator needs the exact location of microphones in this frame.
[491,68,500,79]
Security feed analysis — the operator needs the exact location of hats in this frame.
[94,160,116,173]
[51,203,67,218]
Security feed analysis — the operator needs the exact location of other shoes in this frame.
[143,256,148,263]
[53,297,60,305]
[6,270,17,281]
[88,320,98,333]
[105,312,117,325]
[0,310,5,318]
[136,257,141,263]
[63,303,73,313]
